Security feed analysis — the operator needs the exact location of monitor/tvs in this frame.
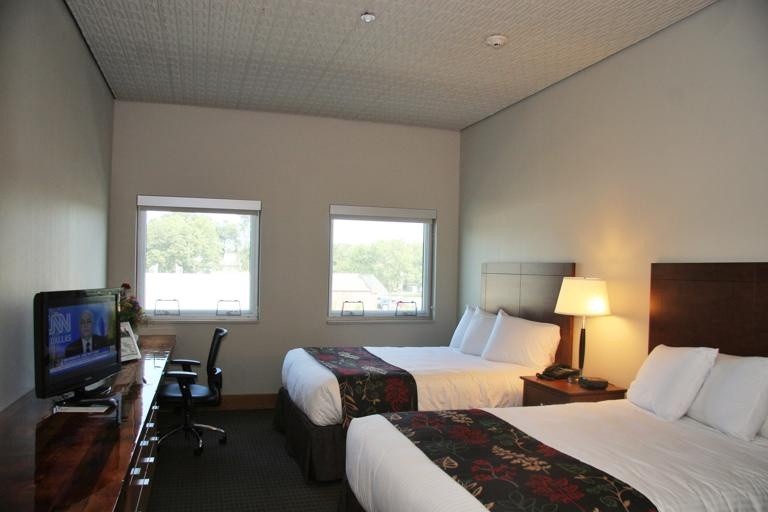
[32,287,122,400]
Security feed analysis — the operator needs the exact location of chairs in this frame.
[157,327,228,456]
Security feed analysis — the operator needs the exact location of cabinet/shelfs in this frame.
[0,334,177,512]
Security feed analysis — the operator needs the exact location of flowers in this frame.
[118,283,143,328]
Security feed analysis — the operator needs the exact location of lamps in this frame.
[554,277,612,384]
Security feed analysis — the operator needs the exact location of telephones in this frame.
[543,364,579,379]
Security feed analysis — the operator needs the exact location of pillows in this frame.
[458,305,497,356]
[686,354,768,442]
[449,305,476,349]
[757,417,768,440]
[482,310,560,367]
[624,345,719,423]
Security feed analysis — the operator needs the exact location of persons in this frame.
[64,310,110,357]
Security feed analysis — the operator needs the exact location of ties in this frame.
[86,342,90,352]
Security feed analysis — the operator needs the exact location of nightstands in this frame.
[520,376,627,407]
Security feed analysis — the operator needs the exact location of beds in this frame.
[276,261,576,485]
[337,262,768,512]
